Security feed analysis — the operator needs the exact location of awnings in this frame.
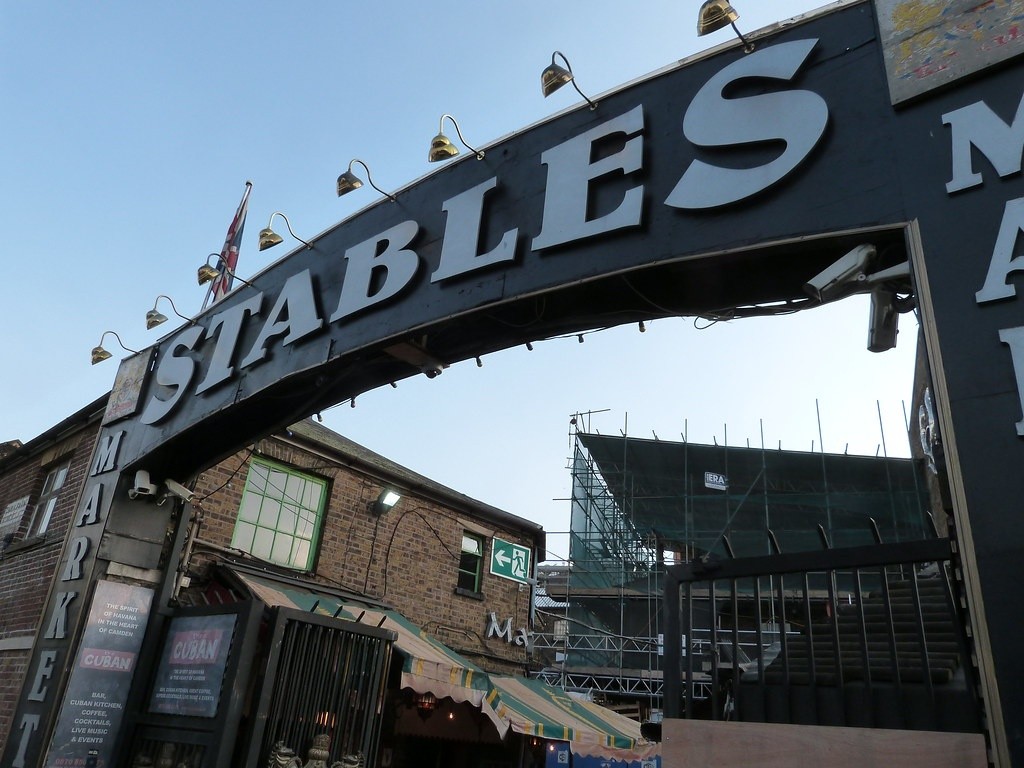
[481,673,661,761]
[236,568,510,735]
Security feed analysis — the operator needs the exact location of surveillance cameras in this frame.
[805,245,878,303]
[867,289,899,354]
[135,470,150,495]
[165,479,196,503]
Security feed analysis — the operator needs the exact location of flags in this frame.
[211,195,247,301]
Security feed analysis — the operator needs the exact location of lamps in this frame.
[540,50,598,111]
[259,211,314,251]
[198,253,252,287]
[428,114,485,163]
[370,488,402,519]
[336,159,397,203]
[146,295,197,330]
[92,331,144,365]
[697,0,755,54]
